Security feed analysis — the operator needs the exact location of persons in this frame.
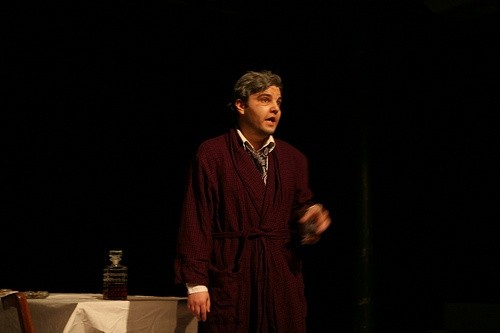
[174,72,331,333]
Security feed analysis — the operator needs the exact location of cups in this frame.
[299,209,319,244]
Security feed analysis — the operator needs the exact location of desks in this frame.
[0,293,198,333]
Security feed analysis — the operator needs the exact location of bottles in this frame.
[103,251,128,300]
